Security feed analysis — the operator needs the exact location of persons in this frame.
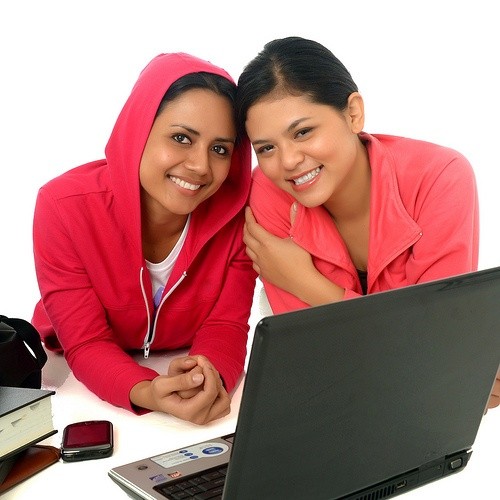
[29,54,259,424]
[235,37,500,417]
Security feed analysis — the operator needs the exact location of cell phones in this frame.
[60,420,113,463]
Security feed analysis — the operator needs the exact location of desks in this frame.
[0,344,500,500]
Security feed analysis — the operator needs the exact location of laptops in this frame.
[110,265,500,500]
